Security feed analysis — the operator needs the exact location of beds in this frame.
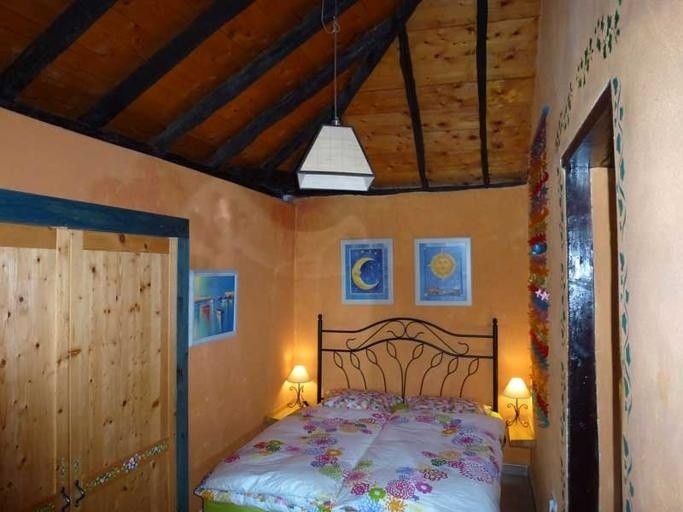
[193,314,506,512]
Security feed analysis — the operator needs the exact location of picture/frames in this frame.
[188,270,238,346]
[340,237,472,307]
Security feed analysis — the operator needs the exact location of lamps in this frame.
[295,1,375,192]
[502,377,530,428]
[287,366,311,408]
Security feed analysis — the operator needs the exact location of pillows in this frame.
[320,388,480,416]
